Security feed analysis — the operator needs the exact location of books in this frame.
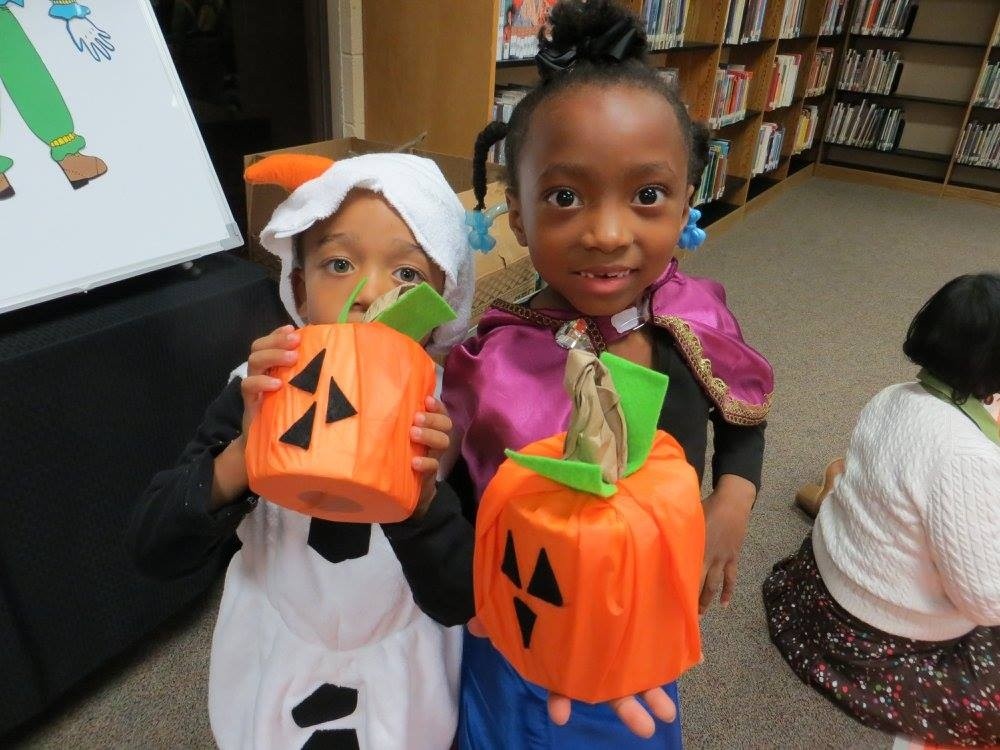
[954,28,1000,172]
[825,0,920,155]
[485,0,563,167]
[642,0,849,210]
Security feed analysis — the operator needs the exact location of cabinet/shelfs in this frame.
[743,0,828,207]
[811,0,998,191]
[943,19,1000,192]
[784,0,857,179]
[688,0,785,216]
[360,0,730,231]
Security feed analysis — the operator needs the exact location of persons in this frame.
[123,152,486,749]
[437,0,778,750]
[764,272,999,750]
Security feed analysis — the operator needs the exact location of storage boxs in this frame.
[242,137,537,342]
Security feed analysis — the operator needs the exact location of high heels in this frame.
[797,457,847,516]
